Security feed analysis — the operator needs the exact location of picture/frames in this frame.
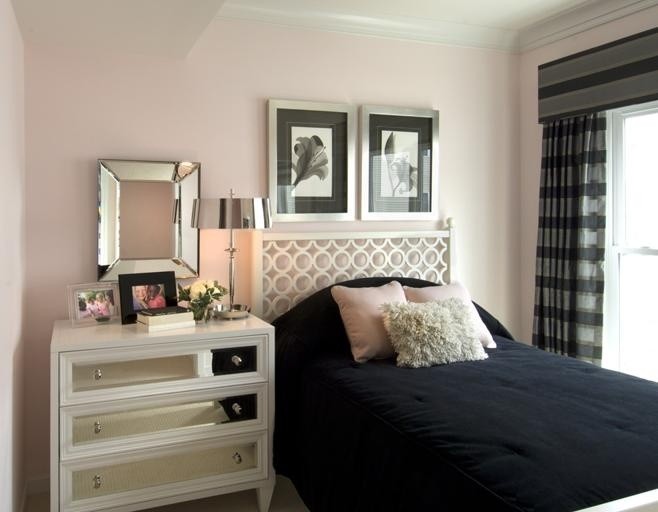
[267,97,440,224]
[65,270,178,329]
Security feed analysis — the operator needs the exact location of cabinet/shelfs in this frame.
[48,328,276,512]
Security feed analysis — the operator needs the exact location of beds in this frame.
[249,218,658,512]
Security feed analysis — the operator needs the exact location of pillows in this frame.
[327,279,496,368]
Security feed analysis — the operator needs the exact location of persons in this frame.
[143,284,167,309]
[83,291,114,316]
[133,285,148,310]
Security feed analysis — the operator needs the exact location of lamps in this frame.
[189,188,272,322]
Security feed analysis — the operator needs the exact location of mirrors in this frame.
[96,156,202,285]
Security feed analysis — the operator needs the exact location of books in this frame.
[135,305,195,325]
[134,320,196,330]
